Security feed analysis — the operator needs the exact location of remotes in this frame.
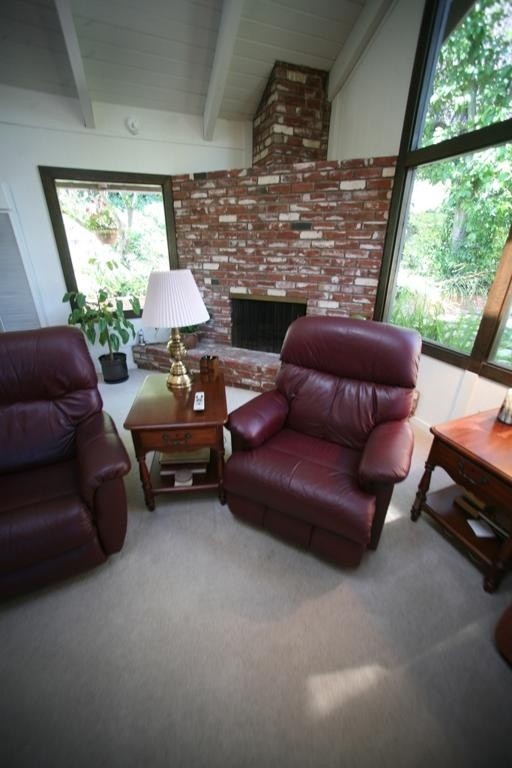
[193,391,205,411]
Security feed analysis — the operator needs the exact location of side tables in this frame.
[123,373,229,512]
[410,407,512,595]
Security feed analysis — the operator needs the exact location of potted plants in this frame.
[61,257,140,385]
[85,207,119,245]
[177,325,201,349]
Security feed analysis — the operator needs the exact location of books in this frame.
[453,487,510,543]
[160,463,208,477]
[158,448,211,464]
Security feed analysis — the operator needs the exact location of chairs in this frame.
[223,314,423,570]
[0,325,132,602]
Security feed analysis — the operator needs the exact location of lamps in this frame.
[140,269,211,390]
